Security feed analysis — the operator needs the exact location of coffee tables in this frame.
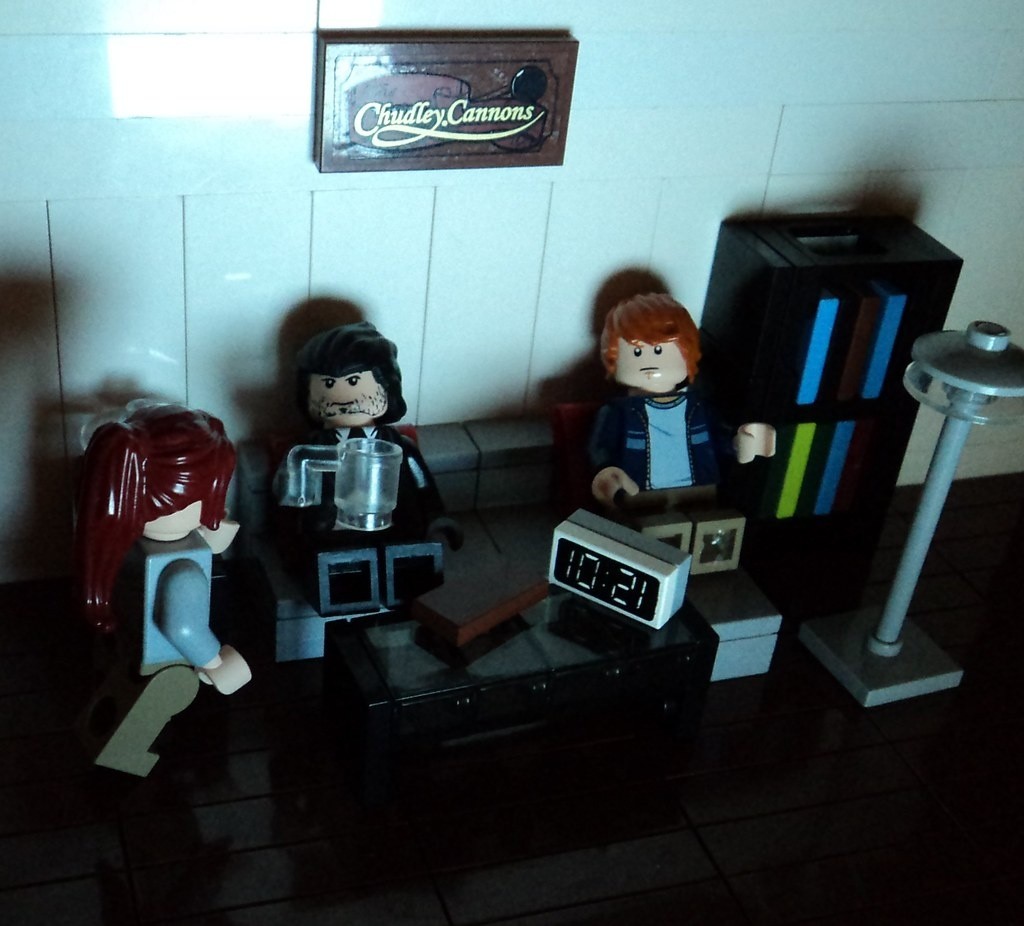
[327,589,718,849]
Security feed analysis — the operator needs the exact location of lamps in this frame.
[800,321,1024,708]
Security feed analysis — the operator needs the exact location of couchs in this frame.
[228,412,784,687]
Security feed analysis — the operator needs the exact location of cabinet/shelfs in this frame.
[691,213,968,589]
